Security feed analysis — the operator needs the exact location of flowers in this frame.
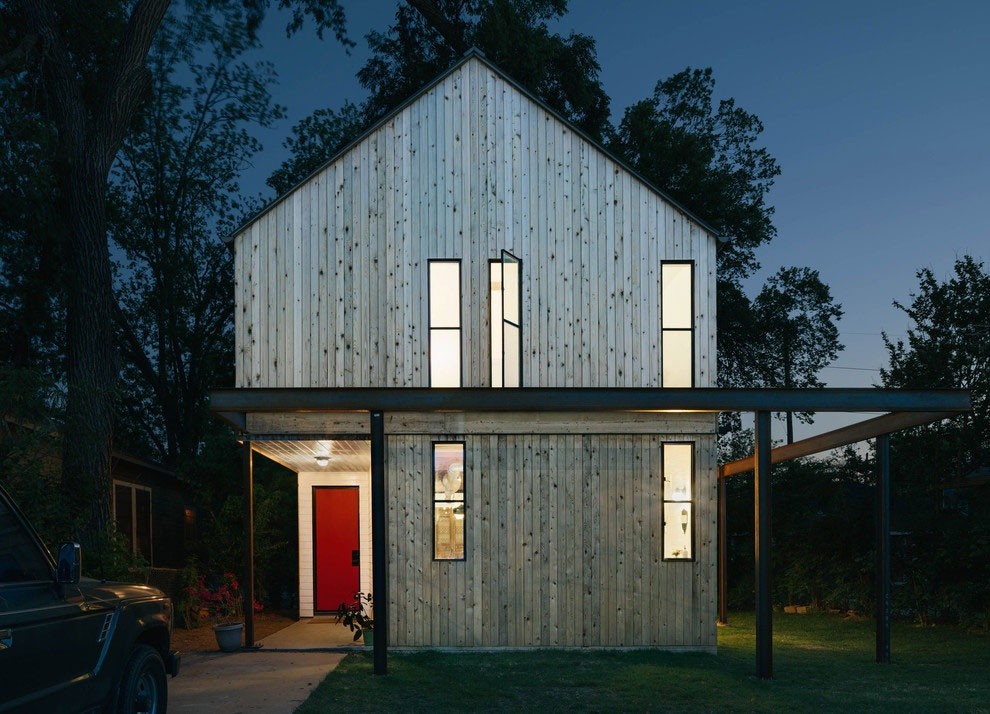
[191,571,266,626]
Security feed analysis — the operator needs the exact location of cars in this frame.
[0,486,182,714]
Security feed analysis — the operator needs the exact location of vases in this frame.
[212,621,245,652]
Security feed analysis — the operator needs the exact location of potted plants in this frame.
[335,590,374,646]
